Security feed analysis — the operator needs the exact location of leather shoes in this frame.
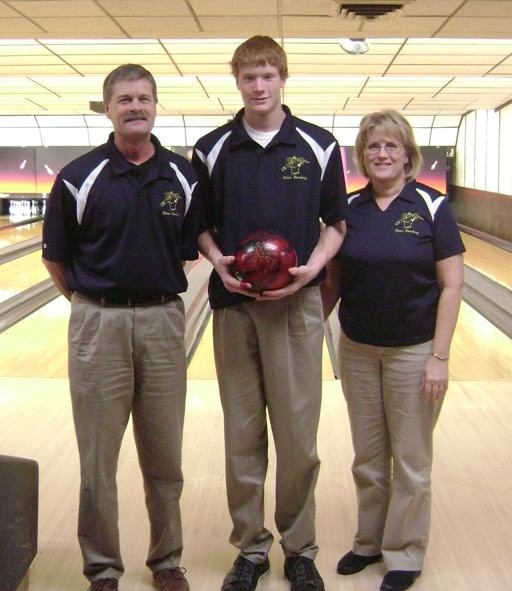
[284,556,325,591]
[336,550,383,574]
[380,570,421,591]
[221,552,270,591]
[86,578,118,591]
[152,567,189,591]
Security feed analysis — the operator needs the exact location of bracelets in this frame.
[431,350,450,364]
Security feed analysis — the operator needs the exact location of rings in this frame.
[238,281,248,291]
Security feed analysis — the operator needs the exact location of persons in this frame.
[39,63,202,591]
[317,107,469,589]
[191,32,350,591]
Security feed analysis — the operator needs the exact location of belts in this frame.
[96,294,176,307]
